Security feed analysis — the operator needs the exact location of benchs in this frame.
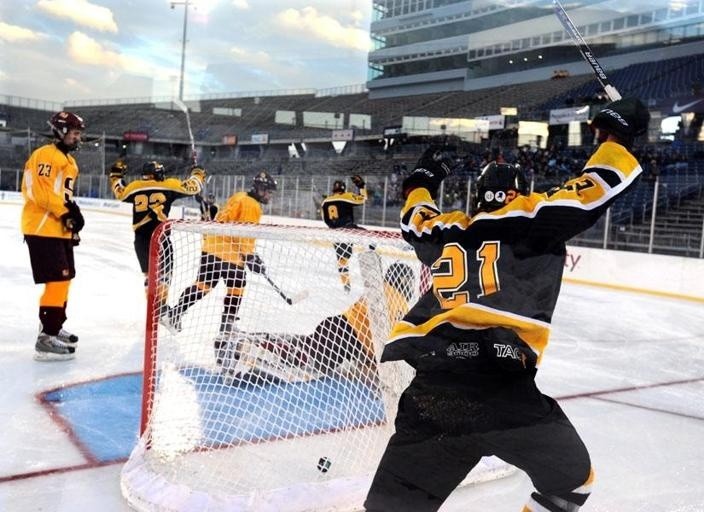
[0,50,704,261]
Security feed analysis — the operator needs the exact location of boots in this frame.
[38,321,79,344]
[34,332,75,356]
[160,305,183,332]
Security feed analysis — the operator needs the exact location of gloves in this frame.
[108,158,129,180]
[351,174,366,188]
[592,94,651,146]
[401,143,459,202]
[241,254,266,274]
[59,210,85,232]
[190,165,208,183]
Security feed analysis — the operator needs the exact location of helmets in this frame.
[47,110,87,135]
[141,161,166,177]
[473,160,528,210]
[331,180,345,192]
[253,170,277,191]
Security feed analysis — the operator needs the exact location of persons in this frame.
[322,174,382,293]
[367,122,687,208]
[363,95,650,512]
[110,160,206,318]
[167,170,277,366]
[214,263,416,385]
[195,192,218,222]
[22,111,86,354]
[550,70,569,81]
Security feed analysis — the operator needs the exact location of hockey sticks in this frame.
[261,270,309,305]
[172,98,198,166]
